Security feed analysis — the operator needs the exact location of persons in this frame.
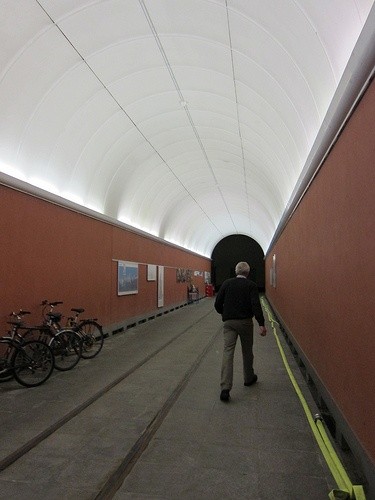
[214,263,267,403]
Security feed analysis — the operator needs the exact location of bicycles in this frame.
[0,320,54,387]
[0,310,84,383]
[19,300,104,370]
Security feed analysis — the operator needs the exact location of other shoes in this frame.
[244,374,257,386]
[220,390,231,402]
